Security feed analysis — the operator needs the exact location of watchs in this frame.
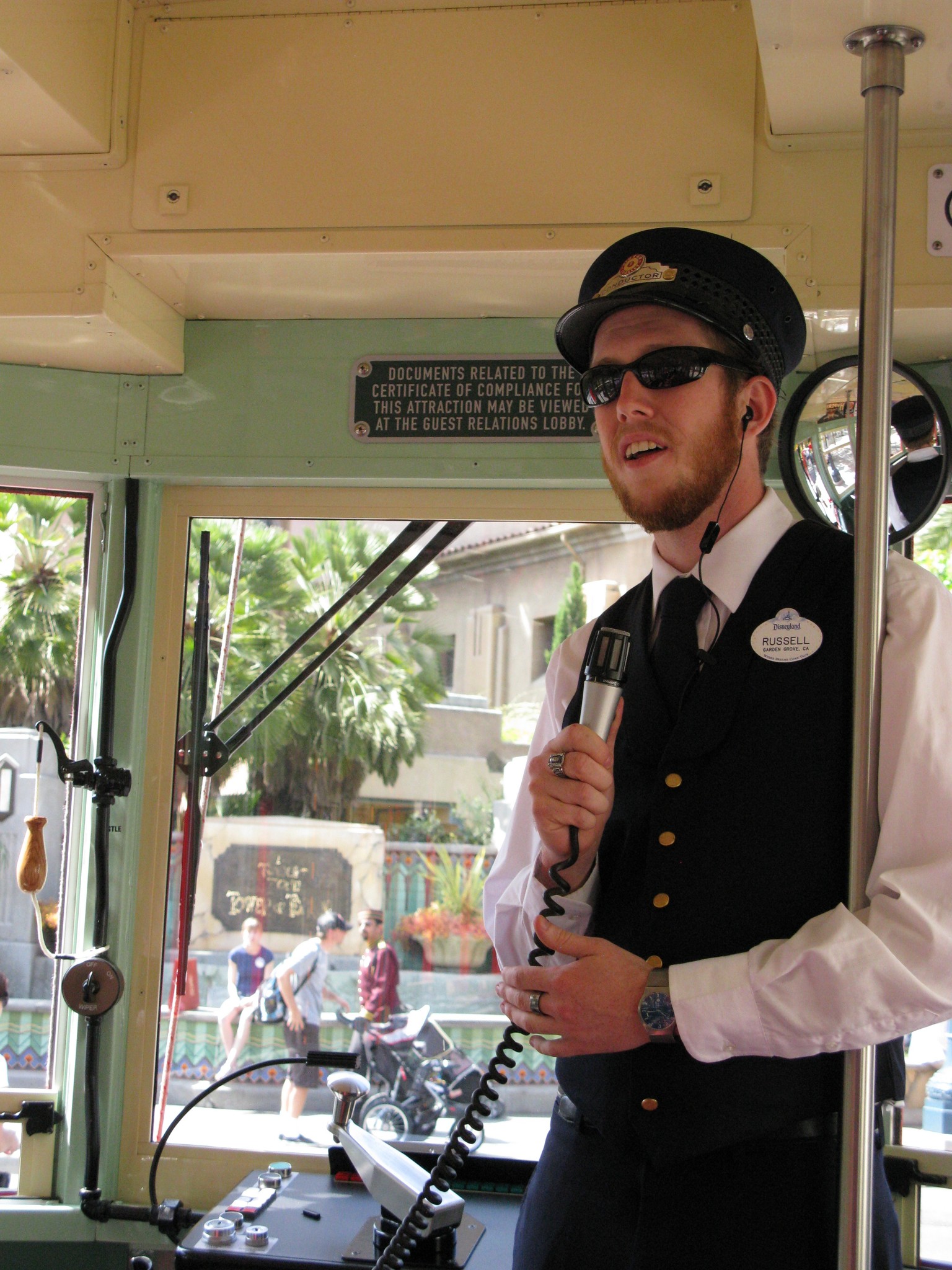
[637,969,676,1044]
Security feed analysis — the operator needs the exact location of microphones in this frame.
[578,622,635,739]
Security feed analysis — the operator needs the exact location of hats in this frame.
[891,395,934,429]
[317,910,353,931]
[554,227,808,399]
[357,910,384,922]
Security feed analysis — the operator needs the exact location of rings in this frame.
[548,751,568,779]
[529,990,548,1016]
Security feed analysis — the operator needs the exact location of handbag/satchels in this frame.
[258,976,287,1024]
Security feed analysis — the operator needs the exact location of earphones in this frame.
[732,402,756,432]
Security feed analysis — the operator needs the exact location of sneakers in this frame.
[279,1134,321,1148]
[214,1065,231,1081]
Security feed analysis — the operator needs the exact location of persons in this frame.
[273,911,353,1143]
[213,916,274,1084]
[479,224,952,1270]
[348,909,400,1055]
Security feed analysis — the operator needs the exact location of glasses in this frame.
[578,345,769,408]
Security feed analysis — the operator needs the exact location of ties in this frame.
[649,574,712,703]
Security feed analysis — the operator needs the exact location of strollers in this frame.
[335,1004,507,1153]
